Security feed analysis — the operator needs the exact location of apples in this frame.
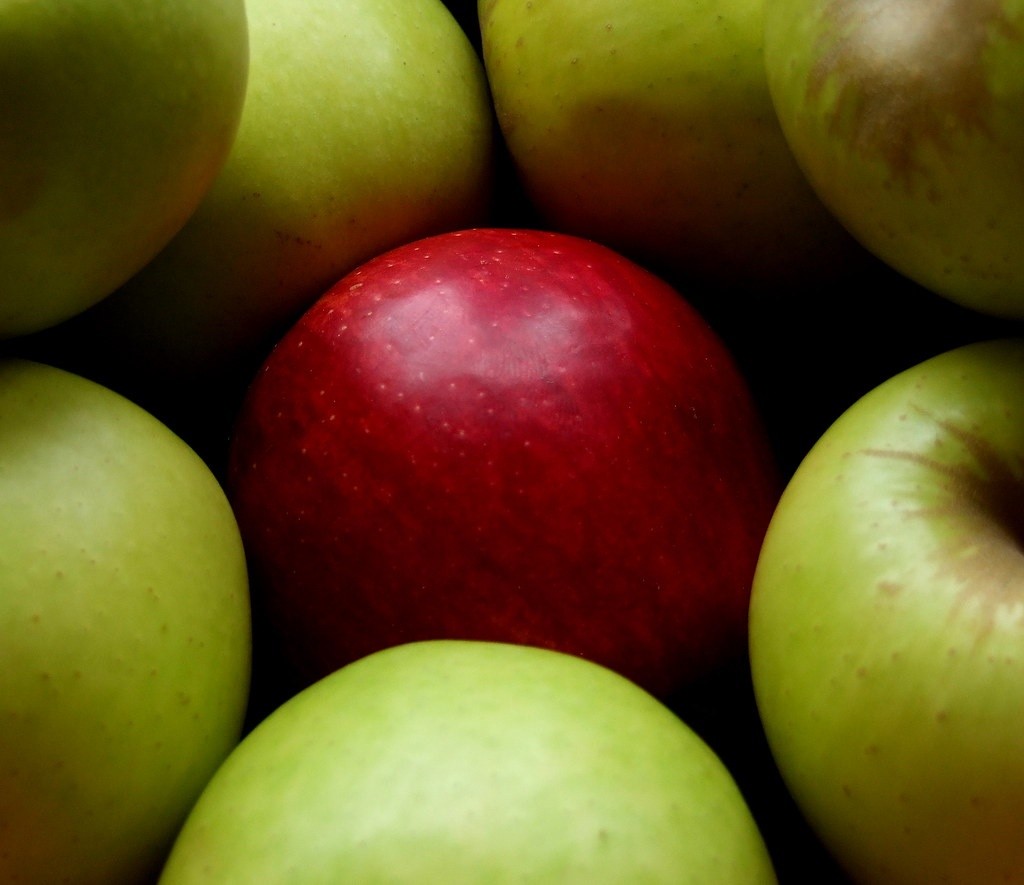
[0,0,1024,885]
